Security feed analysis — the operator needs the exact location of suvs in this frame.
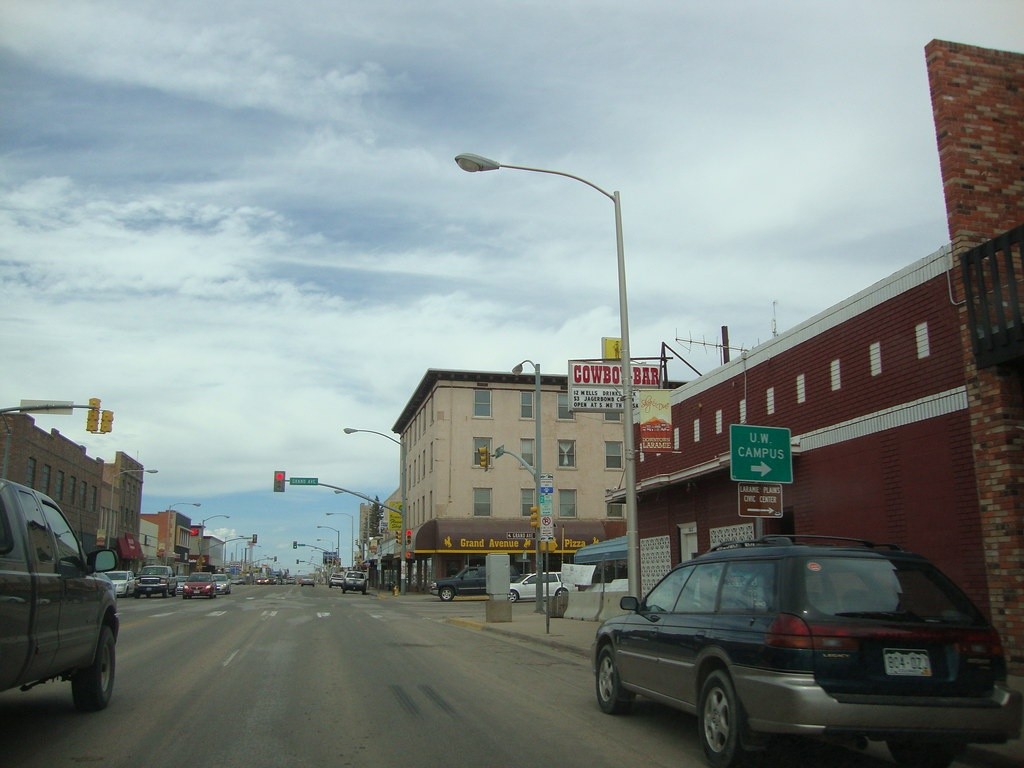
[430,567,524,603]
[137,565,180,599]
[342,571,369,595]
[329,573,344,588]
[0,478,122,708]
[591,531,1015,767]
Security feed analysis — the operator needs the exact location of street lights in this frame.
[165,503,205,565]
[317,538,335,571]
[333,487,370,591]
[509,359,547,614]
[327,512,353,571]
[199,514,231,572]
[343,427,409,596]
[454,156,645,612]
[107,468,158,555]
[317,525,340,570]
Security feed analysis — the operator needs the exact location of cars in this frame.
[184,572,219,597]
[106,570,137,597]
[235,576,316,588]
[506,571,576,604]
[176,574,195,595]
[213,574,236,595]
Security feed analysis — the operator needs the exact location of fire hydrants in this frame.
[393,586,400,596]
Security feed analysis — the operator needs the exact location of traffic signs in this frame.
[729,423,799,489]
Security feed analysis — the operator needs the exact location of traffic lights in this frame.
[538,541,547,553]
[735,481,784,520]
[478,445,489,472]
[274,471,286,493]
[395,530,402,544]
[529,504,539,529]
[405,530,413,547]
[296,559,300,564]
[292,541,298,549]
